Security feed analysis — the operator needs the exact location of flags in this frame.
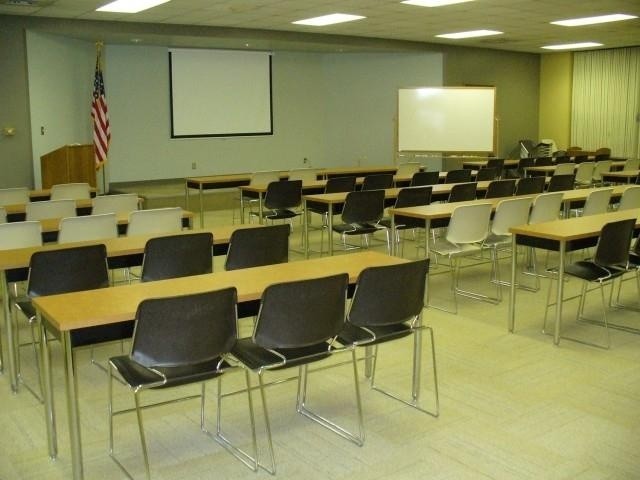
[90,52,111,169]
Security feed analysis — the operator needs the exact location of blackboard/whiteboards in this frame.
[396,86,497,155]
